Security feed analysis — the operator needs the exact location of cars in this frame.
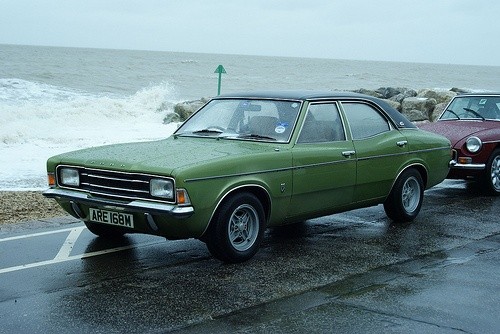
[41,89,458,264]
[416,91,500,195]
